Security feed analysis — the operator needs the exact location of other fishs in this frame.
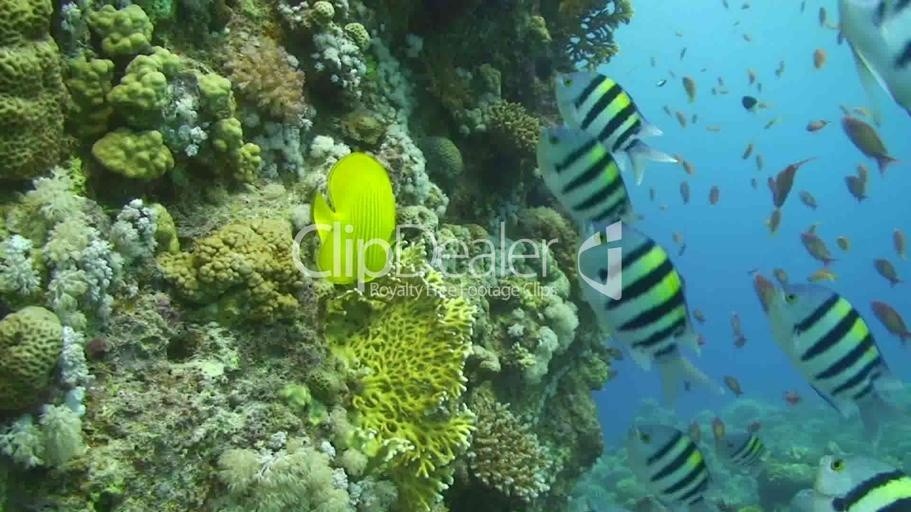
[312,151,396,285]
[535,0,910,512]
